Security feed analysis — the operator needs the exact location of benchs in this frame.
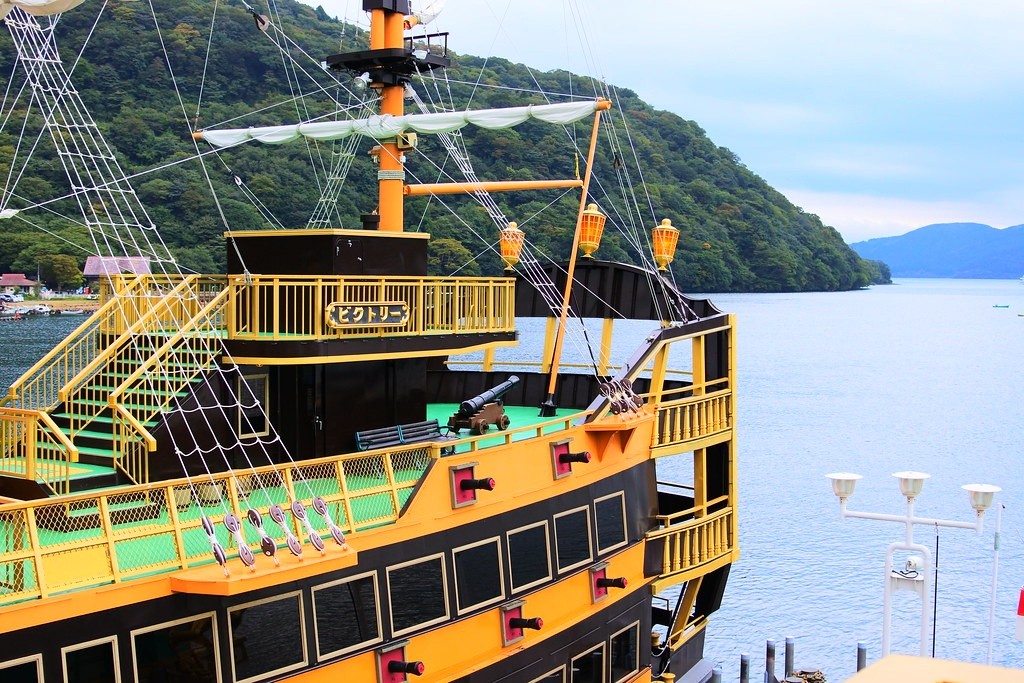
[355,418,461,479]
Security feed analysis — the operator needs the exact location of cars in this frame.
[0,294,24,303]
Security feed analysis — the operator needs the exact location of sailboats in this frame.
[0,1,739,683]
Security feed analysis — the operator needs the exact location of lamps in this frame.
[353,72,373,89]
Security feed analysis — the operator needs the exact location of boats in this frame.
[0,304,85,322]
